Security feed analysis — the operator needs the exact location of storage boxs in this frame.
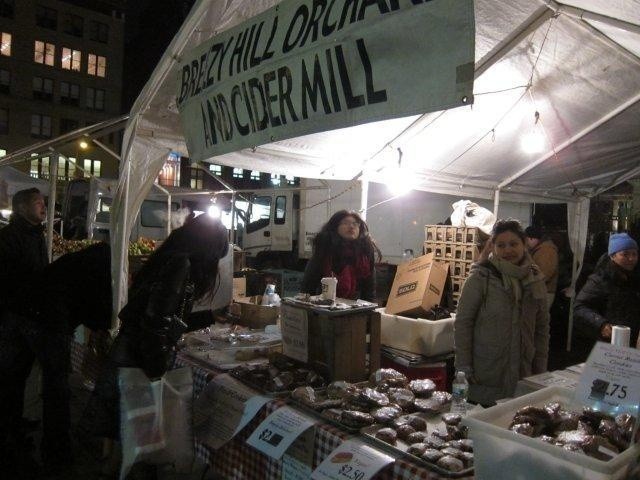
[376,251,458,389]
[234,269,304,324]
[462,385,640,479]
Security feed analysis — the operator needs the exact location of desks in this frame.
[164,351,474,480]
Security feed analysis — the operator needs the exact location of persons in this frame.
[571,230,639,361]
[83,210,229,480]
[1,187,50,480]
[294,207,377,328]
[525,224,558,314]
[7,237,114,480]
[452,220,550,409]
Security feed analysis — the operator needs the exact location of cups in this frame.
[262,283,275,305]
[320,276,338,301]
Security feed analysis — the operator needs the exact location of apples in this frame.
[129,236,155,255]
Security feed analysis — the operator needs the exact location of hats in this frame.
[523,225,543,240]
[608,232,639,256]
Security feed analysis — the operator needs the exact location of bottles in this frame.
[450,371,470,416]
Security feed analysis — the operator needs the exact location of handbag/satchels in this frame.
[117,298,189,353]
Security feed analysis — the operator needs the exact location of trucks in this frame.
[240,176,532,282]
[63,176,228,247]
